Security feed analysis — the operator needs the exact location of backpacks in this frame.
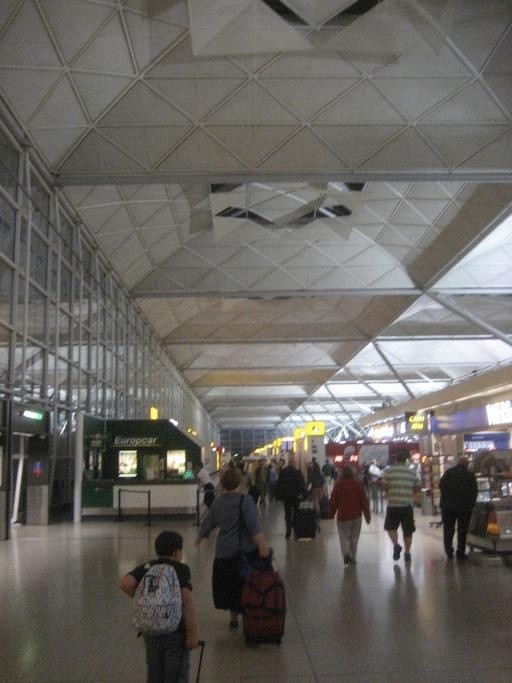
[130,562,183,637]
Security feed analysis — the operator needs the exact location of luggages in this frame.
[292,494,318,541]
[319,496,335,518]
[239,546,285,646]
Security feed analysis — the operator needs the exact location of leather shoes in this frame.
[447,550,468,560]
[344,553,357,564]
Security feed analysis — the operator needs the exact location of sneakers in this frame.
[393,544,402,560]
[230,620,239,631]
[405,552,411,561]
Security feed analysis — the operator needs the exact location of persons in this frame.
[118,530,199,683]
[274,454,306,539]
[193,469,269,628]
[491,452,512,479]
[194,462,214,510]
[219,455,383,522]
[438,455,478,560]
[326,465,370,565]
[379,448,422,562]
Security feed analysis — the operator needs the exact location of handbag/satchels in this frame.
[232,551,273,573]
